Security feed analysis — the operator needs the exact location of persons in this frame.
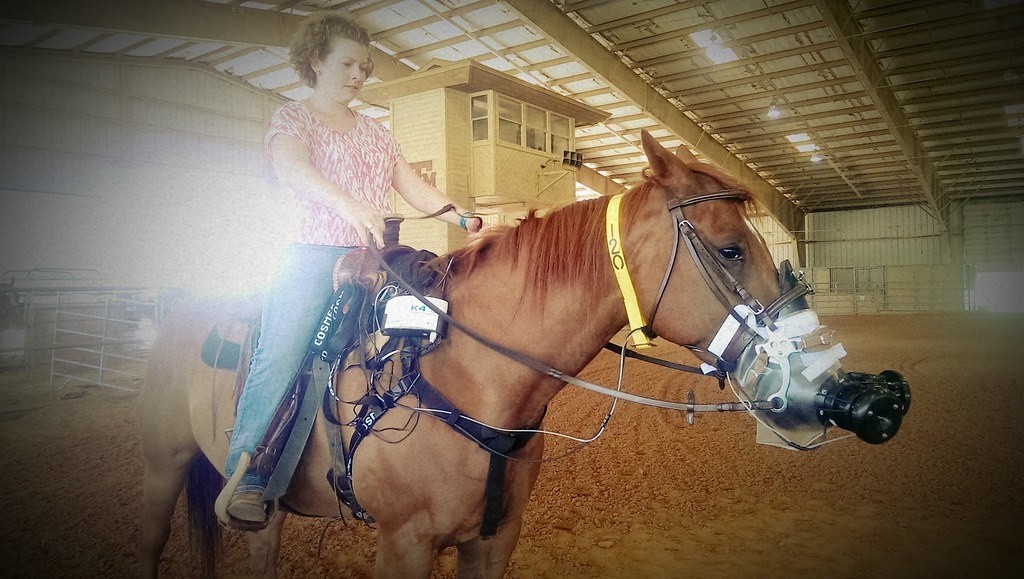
[227,12,486,523]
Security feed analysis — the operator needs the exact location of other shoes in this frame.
[226,491,266,522]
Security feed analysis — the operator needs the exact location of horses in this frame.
[140,128,842,579]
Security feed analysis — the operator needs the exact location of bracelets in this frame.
[459,212,471,231]
